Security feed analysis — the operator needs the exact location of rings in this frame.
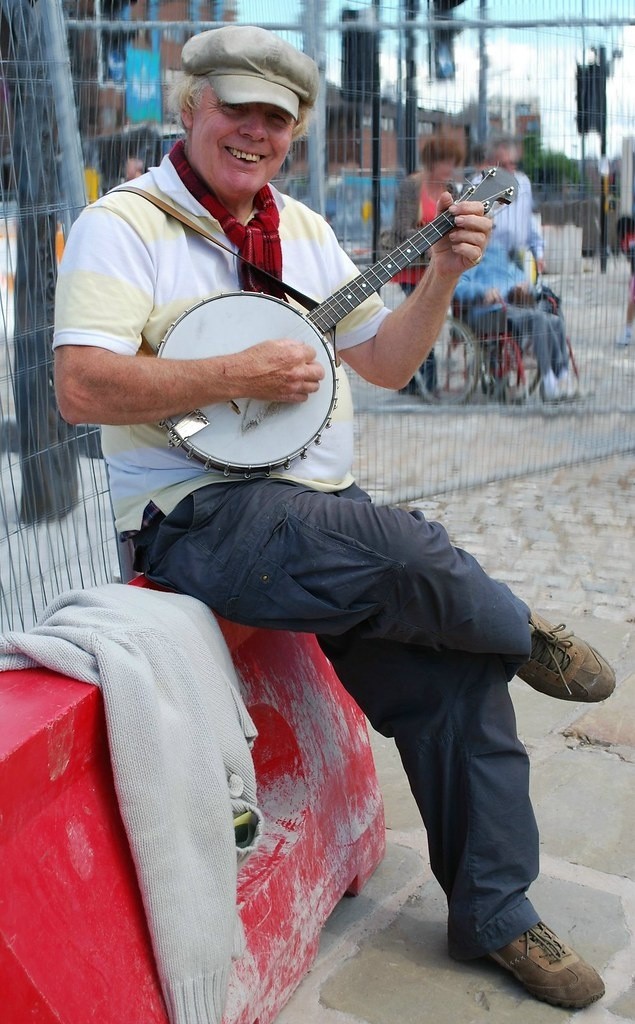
[472,252,482,266]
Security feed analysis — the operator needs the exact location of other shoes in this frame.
[540,369,574,402]
[488,920,605,1008]
[515,609,615,703]
[617,333,631,346]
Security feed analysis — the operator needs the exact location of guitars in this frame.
[154,163,524,479]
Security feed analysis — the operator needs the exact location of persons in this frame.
[619,156,635,347]
[125,158,143,182]
[51,26,617,1008]
[390,137,580,402]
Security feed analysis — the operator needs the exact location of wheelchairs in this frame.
[411,255,580,404]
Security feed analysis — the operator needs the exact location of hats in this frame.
[181,25,319,122]
